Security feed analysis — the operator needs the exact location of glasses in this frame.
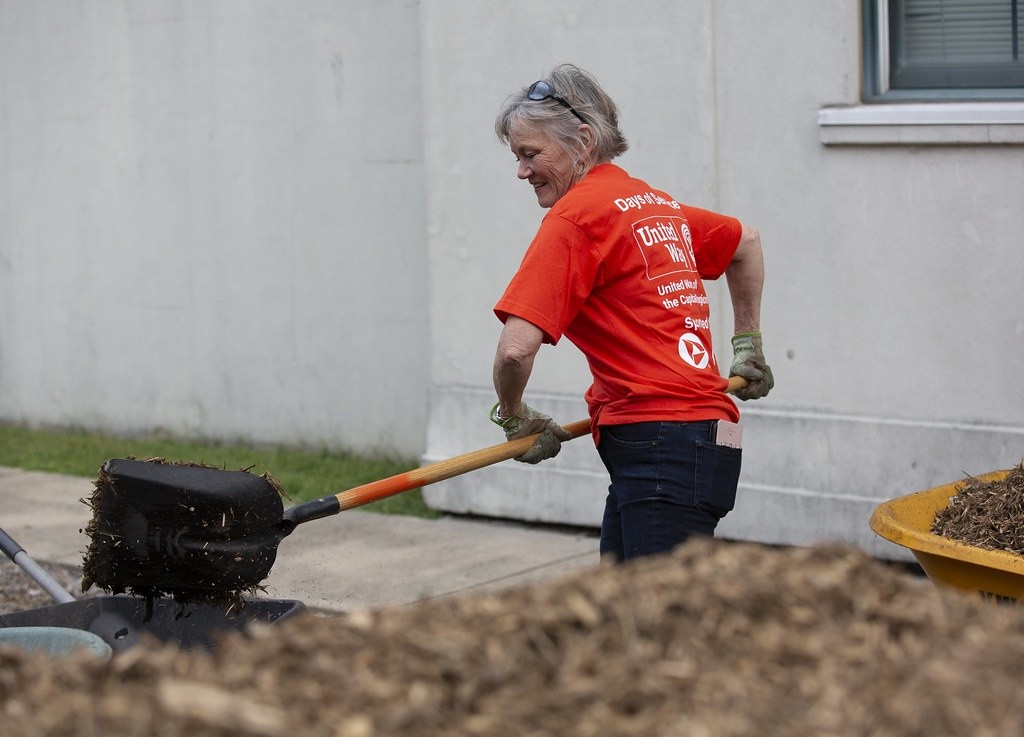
[526,81,587,124]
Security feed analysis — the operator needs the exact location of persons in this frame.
[489,62,776,565]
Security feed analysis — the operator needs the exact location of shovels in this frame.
[83,369,761,596]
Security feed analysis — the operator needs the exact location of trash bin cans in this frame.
[868,468,1022,609]
[0,589,307,694]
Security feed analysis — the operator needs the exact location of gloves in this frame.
[490,401,573,465]
[729,331,774,401]
